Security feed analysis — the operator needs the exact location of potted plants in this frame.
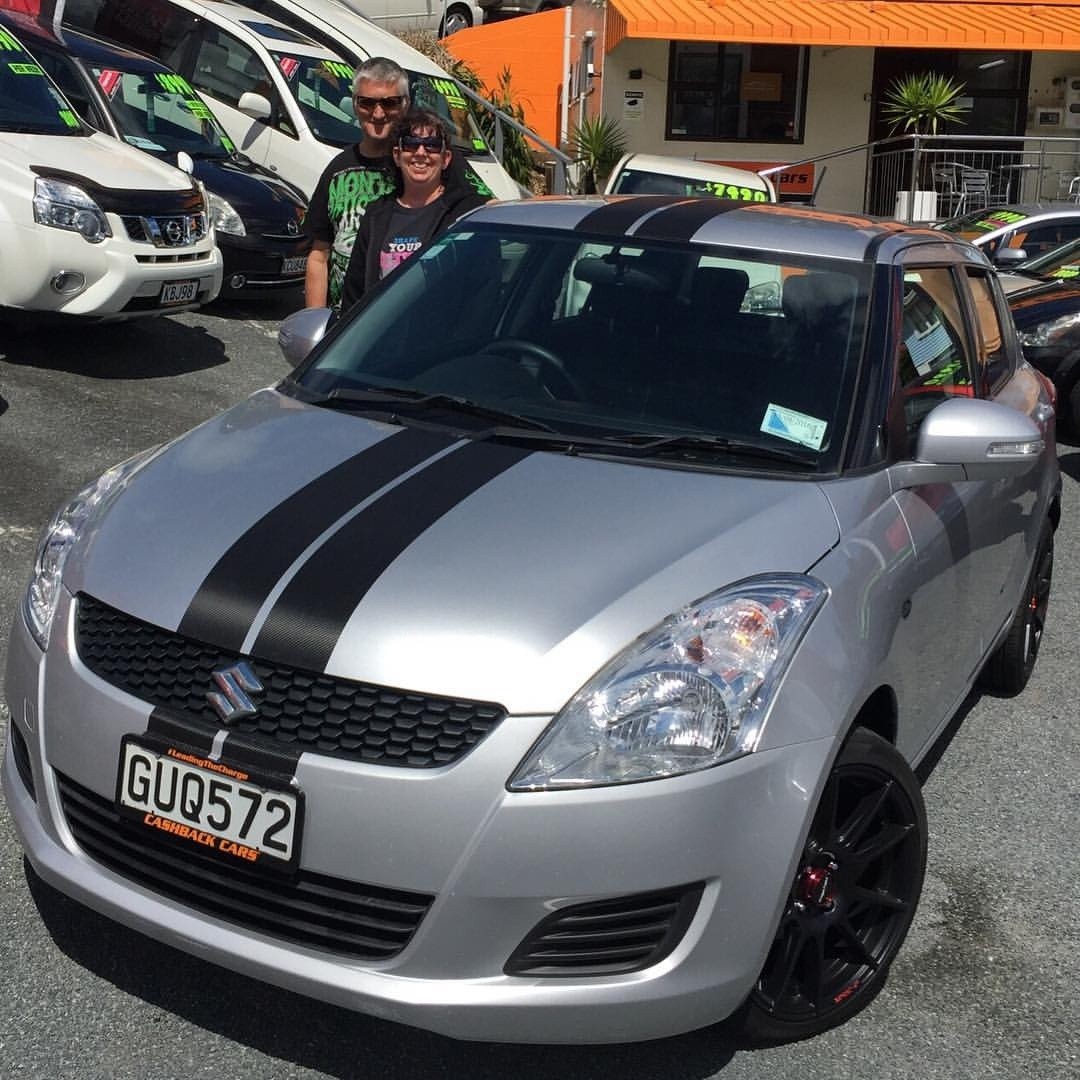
[879,72,973,228]
[567,112,631,192]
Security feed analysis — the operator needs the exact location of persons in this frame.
[341,108,501,371]
[573,253,705,306]
[304,57,503,309]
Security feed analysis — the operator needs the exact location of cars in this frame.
[0,23,224,324]
[895,203,1079,448]
[438,0,575,41]
[0,190,1064,1047]
[0,6,309,290]
[563,153,783,317]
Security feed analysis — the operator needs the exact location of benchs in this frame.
[696,267,797,361]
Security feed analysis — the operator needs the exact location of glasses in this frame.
[354,93,407,113]
[398,134,447,153]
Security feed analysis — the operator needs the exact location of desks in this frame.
[998,164,1052,204]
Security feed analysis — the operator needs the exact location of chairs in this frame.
[929,162,1011,218]
[769,273,855,324]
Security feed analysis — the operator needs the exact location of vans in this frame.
[0,0,529,260]
